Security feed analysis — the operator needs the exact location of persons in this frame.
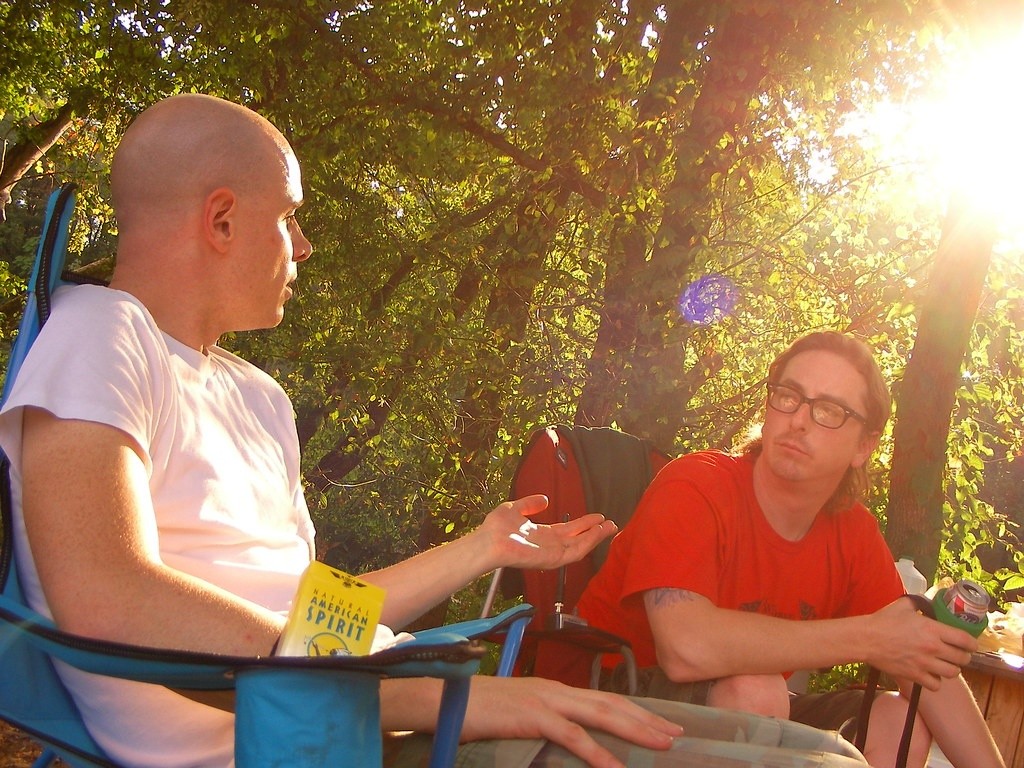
[573,331,1006,768]
[0,95,868,768]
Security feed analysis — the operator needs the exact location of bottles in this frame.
[894,559,927,595]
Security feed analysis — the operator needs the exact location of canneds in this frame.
[942,580,991,625]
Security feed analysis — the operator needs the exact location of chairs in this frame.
[478,428,675,696]
[0,179,531,768]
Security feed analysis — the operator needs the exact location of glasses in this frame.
[766,382,869,430]
